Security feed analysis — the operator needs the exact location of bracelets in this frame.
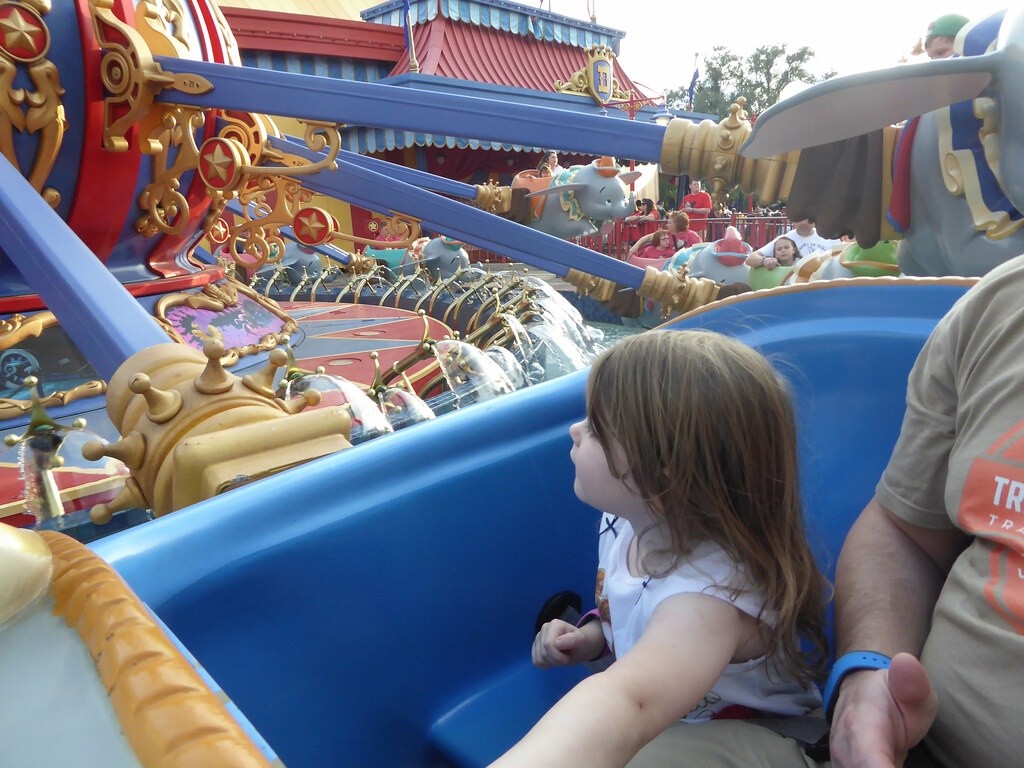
[761,257,766,267]
[823,650,892,726]
[692,208,694,212]
[576,608,610,661]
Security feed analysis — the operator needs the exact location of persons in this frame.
[822,255,1024,768]
[525,150,563,180]
[773,236,802,266]
[485,329,835,768]
[745,218,851,281]
[626,211,701,263]
[640,231,676,258]
[911,14,969,60]
[625,199,659,221]
[679,178,712,242]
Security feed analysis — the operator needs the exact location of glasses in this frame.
[660,238,669,241]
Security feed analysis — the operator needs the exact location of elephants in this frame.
[524,157,642,240]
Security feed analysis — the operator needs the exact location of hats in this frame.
[639,198,653,205]
[925,14,970,36]
